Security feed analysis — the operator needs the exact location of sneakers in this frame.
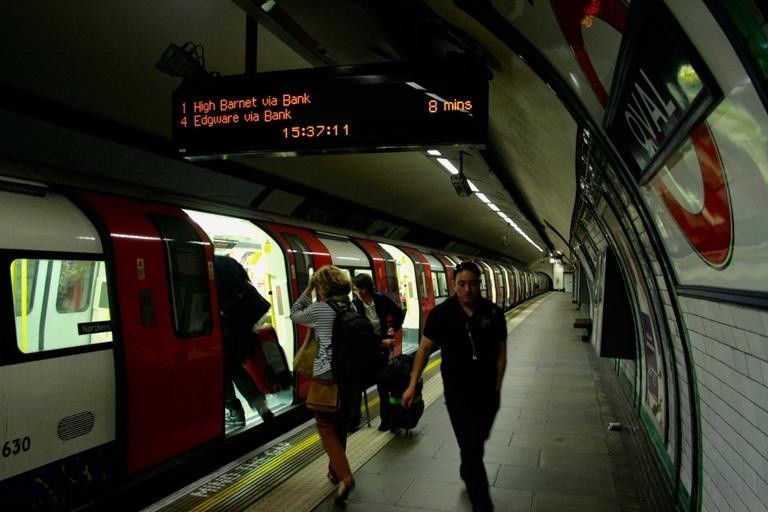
[225,409,245,426]
[348,420,389,434]
[327,470,339,484]
[334,479,356,502]
[259,404,274,421]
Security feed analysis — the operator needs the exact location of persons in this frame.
[400,263,508,512]
[290,263,357,504]
[351,269,405,432]
[213,255,275,429]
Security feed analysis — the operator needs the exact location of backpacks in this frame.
[324,298,377,381]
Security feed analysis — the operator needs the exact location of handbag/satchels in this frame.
[221,280,270,328]
[293,327,324,376]
[306,382,340,413]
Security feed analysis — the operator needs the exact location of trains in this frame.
[1,157,550,512]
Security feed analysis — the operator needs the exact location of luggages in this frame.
[242,327,291,394]
[389,369,424,429]
